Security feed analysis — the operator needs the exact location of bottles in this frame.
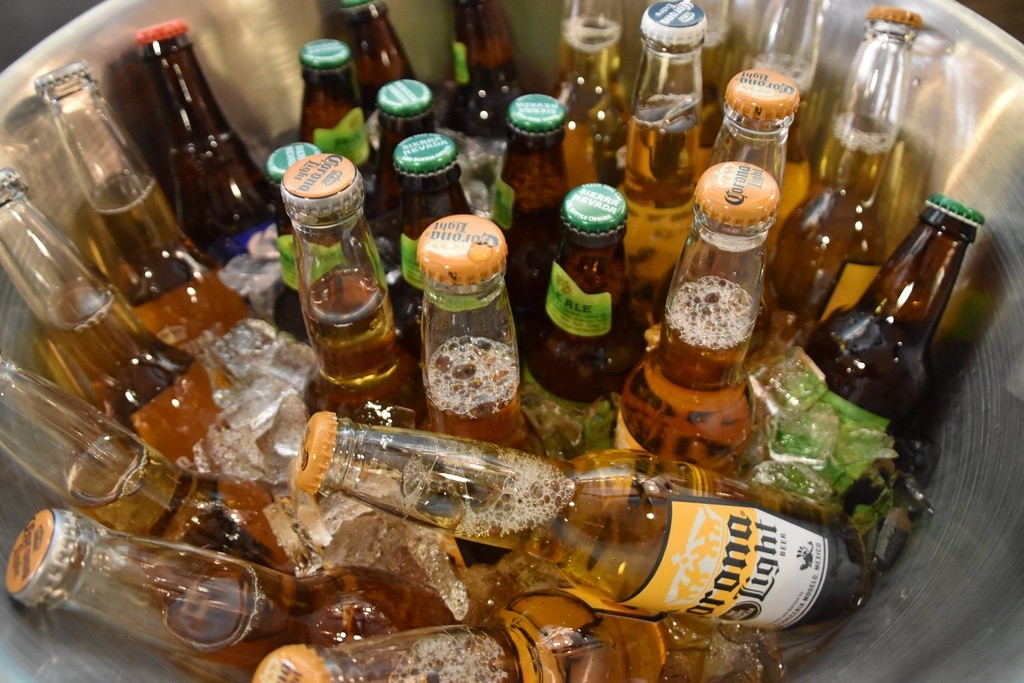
[31,62,264,408]
[0,165,271,492]
[1,507,460,683]
[0,355,299,576]
[241,1,987,681]
[134,22,292,286]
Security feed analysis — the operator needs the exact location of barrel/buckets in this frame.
[0,0,1024,683]
